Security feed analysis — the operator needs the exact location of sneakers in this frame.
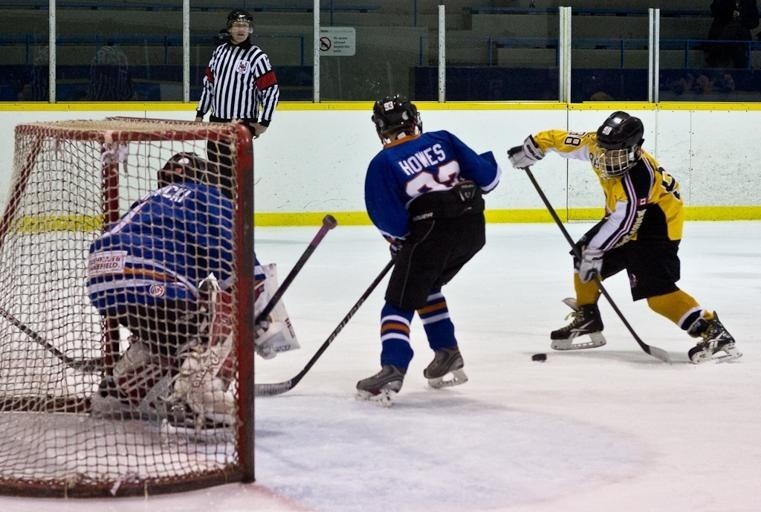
[357,365,405,394]
[167,402,229,429]
[99,376,130,405]
[424,345,463,378]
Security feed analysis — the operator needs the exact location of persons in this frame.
[194,8,279,199]
[85,152,305,447]
[506,110,743,366]
[353,92,500,399]
[706,0,761,69]
[29,19,49,100]
[693,46,738,96]
[83,17,140,101]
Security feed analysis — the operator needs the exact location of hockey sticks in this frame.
[526,166,670,363]
[254,260,397,396]
[0,308,129,370]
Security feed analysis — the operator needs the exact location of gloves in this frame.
[390,244,403,261]
[195,117,203,123]
[507,135,544,169]
[248,122,266,139]
[580,246,604,283]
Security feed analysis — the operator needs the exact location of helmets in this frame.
[592,112,643,178]
[372,95,422,143]
[156,153,219,190]
[226,10,253,34]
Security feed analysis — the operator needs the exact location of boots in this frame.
[687,310,734,359]
[551,299,603,339]
